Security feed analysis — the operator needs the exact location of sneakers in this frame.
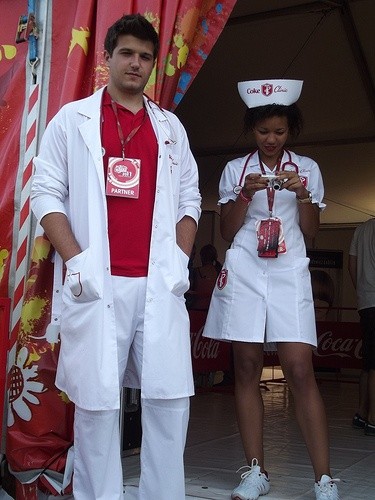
[232,458,270,500]
[313,474,341,500]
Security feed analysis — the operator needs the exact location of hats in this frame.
[238,80,304,108]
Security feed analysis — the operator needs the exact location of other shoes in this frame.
[364,420,375,436]
[352,414,368,428]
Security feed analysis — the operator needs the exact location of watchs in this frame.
[296,191,312,208]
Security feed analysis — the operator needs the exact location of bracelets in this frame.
[239,190,253,202]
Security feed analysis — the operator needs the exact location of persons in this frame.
[348,218,375,437]
[310,270,334,321]
[28,10,202,500]
[202,77,341,500]
[189,244,219,310]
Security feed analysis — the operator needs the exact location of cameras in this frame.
[261,175,284,190]
